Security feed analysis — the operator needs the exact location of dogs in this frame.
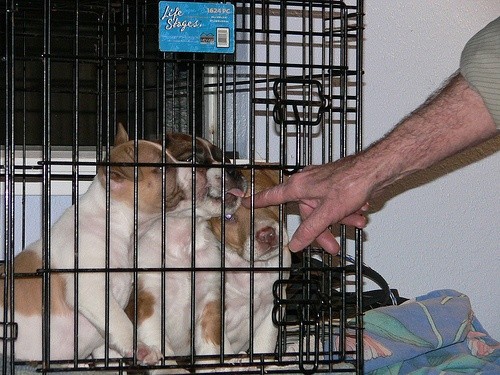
[0,122,292,375]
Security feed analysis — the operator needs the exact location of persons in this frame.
[239,16,500,258]
